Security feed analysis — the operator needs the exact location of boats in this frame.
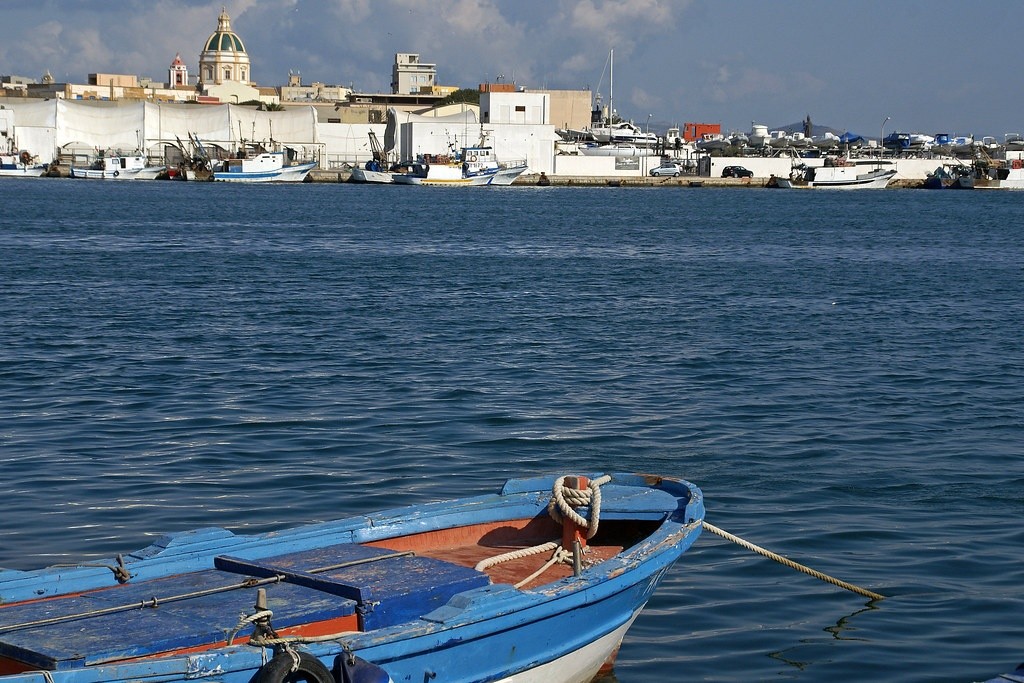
[0,471,705,683]
[766,147,898,188]
[0,104,45,177]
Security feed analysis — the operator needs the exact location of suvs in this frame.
[720,166,754,179]
[649,162,683,177]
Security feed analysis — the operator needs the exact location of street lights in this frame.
[880,115,890,169]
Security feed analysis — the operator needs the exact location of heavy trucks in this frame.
[884,130,1022,149]
[665,122,721,149]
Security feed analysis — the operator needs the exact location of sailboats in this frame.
[39,94,529,186]
[555,48,657,145]
